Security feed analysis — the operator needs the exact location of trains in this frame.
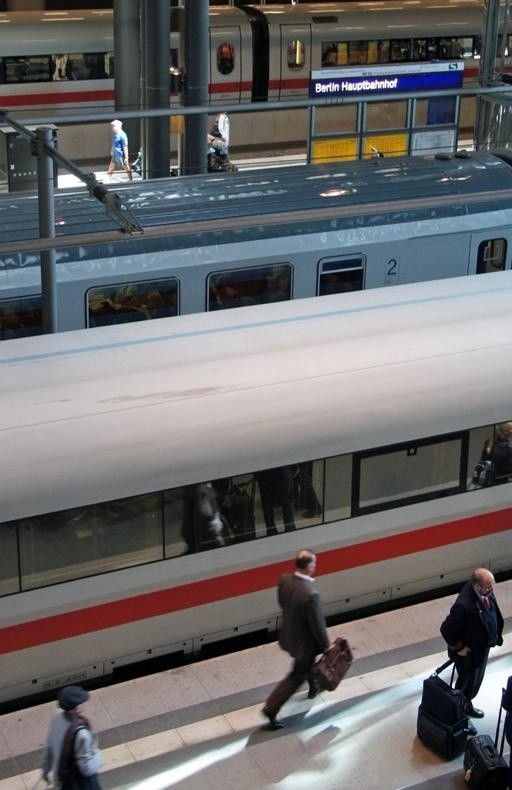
[0,145,511,344]
[0,0,512,120]
[0,0,511,167]
[0,268,512,711]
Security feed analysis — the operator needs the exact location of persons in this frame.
[253,464,327,536]
[0,299,43,338]
[439,566,504,735]
[180,482,229,552]
[106,119,132,181]
[210,266,290,308]
[89,280,177,327]
[207,112,230,173]
[41,685,104,789]
[479,422,512,485]
[261,547,331,731]
[52,55,68,80]
[501,675,511,790]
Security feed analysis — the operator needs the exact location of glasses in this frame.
[479,582,495,588]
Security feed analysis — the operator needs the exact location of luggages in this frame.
[222,476,258,541]
[464,687,510,790]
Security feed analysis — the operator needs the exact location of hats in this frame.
[57,686,90,710]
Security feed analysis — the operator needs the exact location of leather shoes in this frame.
[262,709,284,728]
[308,689,323,698]
[302,508,321,518]
[468,706,484,735]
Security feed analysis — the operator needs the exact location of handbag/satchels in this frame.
[313,637,353,691]
[60,726,102,790]
[417,658,468,759]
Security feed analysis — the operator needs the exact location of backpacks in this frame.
[472,461,495,485]
